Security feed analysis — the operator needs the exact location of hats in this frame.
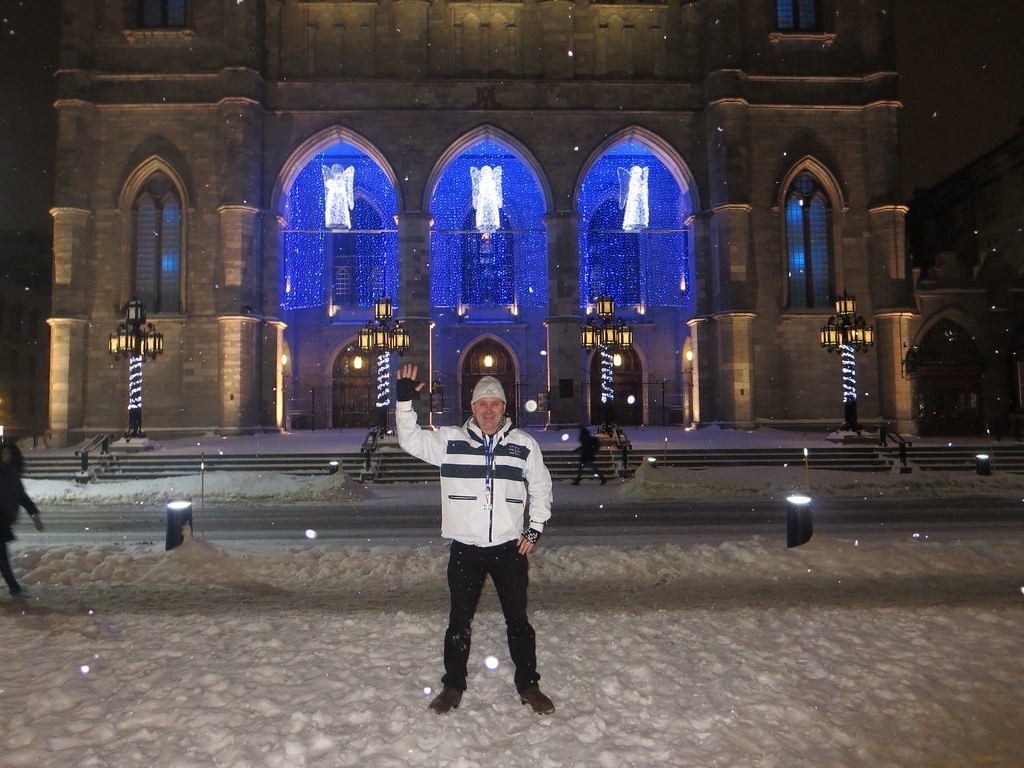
[470,376,506,405]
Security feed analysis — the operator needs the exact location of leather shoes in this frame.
[430,683,462,713]
[516,682,554,713]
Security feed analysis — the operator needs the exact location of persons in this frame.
[394,363,556,718]
[568,423,607,487]
[0,441,45,600]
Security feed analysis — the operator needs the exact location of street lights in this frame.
[356,297,411,437]
[818,288,876,435]
[580,294,634,444]
[106,294,164,439]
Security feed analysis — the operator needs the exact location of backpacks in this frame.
[590,436,600,454]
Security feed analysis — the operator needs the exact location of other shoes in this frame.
[600,476,607,486]
[571,481,580,486]
